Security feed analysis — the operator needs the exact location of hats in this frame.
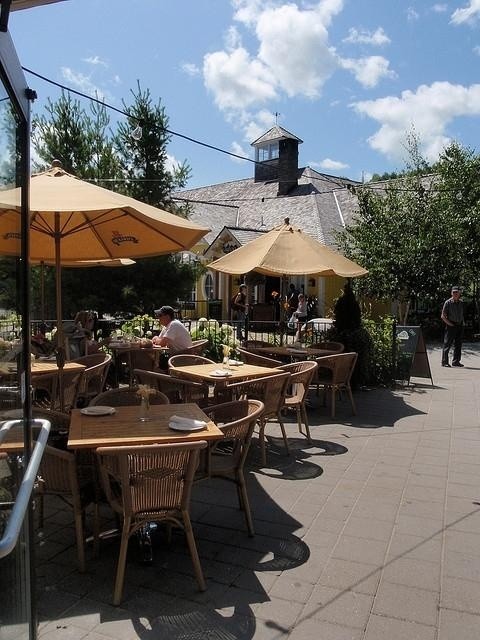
[452,287,458,292]
[154,306,174,315]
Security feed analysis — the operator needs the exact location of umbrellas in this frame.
[0,160,211,413]
[28,255,136,319]
[206,217,369,322]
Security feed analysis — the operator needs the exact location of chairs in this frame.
[201,400,264,538]
[65,353,112,409]
[1,409,104,571]
[306,352,356,420]
[97,441,204,605]
[235,346,288,367]
[133,369,212,466]
[241,340,275,348]
[168,351,215,405]
[309,343,344,359]
[175,339,209,356]
[89,388,169,408]
[215,372,292,466]
[260,362,317,447]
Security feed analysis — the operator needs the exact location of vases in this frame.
[137,396,152,423]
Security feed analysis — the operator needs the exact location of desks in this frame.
[257,346,341,361]
[170,361,286,447]
[68,405,223,570]
[1,362,86,405]
[110,343,170,388]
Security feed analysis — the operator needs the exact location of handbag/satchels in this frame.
[231,295,240,310]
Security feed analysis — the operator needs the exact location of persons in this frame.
[308,278,316,295]
[287,283,300,308]
[440,286,466,367]
[234,284,249,340]
[152,306,192,370]
[73,311,128,386]
[335,284,361,332]
[293,294,307,324]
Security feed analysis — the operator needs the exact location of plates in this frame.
[168,417,204,430]
[79,404,116,416]
[228,362,244,366]
[209,372,230,377]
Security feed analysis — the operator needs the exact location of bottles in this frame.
[139,394,152,421]
[223,356,230,369]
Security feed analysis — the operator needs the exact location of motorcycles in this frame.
[277,293,317,332]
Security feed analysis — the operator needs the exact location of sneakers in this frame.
[452,360,464,367]
[443,361,450,367]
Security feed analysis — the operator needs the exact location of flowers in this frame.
[135,384,157,396]
